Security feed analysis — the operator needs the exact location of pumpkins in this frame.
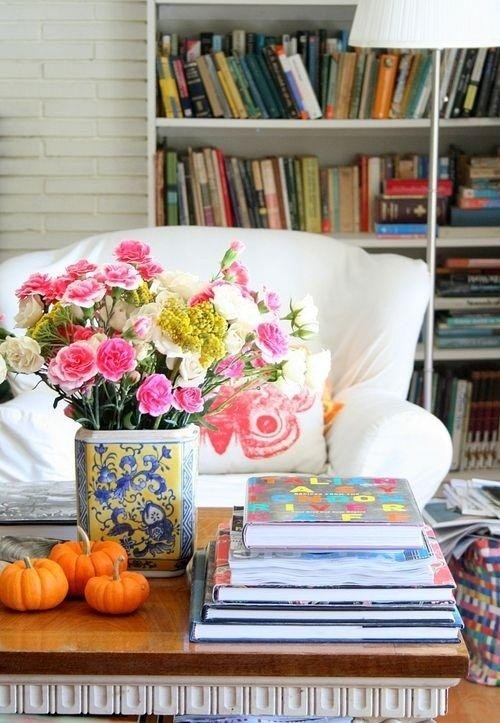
[0,556,69,611]
[49,526,127,597]
[85,556,149,614]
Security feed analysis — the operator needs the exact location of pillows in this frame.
[196,345,331,475]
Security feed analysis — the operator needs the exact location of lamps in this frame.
[347,1,500,415]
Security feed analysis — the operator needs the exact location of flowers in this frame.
[0,240,316,431]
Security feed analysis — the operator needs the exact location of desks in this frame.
[0,508,470,723]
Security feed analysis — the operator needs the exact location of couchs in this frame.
[0,227,453,512]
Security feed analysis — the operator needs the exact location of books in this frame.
[200,540,456,624]
[212,517,457,604]
[190,550,466,644]
[241,473,427,552]
[421,476,500,562]
[226,504,438,585]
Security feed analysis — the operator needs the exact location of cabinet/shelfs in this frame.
[146,0,500,487]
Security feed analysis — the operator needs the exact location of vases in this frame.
[75,427,197,577]
